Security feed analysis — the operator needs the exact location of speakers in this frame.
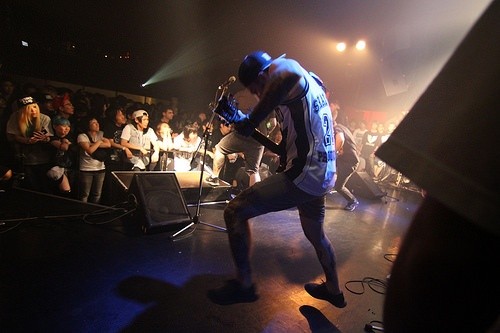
[98,170,233,234]
[345,171,384,200]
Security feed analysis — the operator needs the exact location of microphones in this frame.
[218,76,235,89]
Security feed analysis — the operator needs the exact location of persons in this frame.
[207,53,347,309]
[0,78,283,204]
[346,118,397,173]
[329,103,360,211]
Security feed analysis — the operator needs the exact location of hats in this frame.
[16,95,39,109]
[54,118,70,125]
[52,93,70,112]
[238,51,286,88]
[40,94,53,105]
[132,110,149,119]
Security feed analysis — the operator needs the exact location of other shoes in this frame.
[304,278,345,308]
[207,282,258,305]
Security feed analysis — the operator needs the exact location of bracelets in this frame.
[123,147,126,150]
[246,114,259,128]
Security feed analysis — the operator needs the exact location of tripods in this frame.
[384,185,399,204]
[169,86,230,239]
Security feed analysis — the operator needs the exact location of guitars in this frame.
[212,92,287,173]
[208,101,215,110]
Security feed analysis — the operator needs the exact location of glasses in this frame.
[63,103,71,107]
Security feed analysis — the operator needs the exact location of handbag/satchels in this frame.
[91,147,111,162]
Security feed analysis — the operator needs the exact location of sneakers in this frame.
[344,198,359,211]
[205,175,219,185]
